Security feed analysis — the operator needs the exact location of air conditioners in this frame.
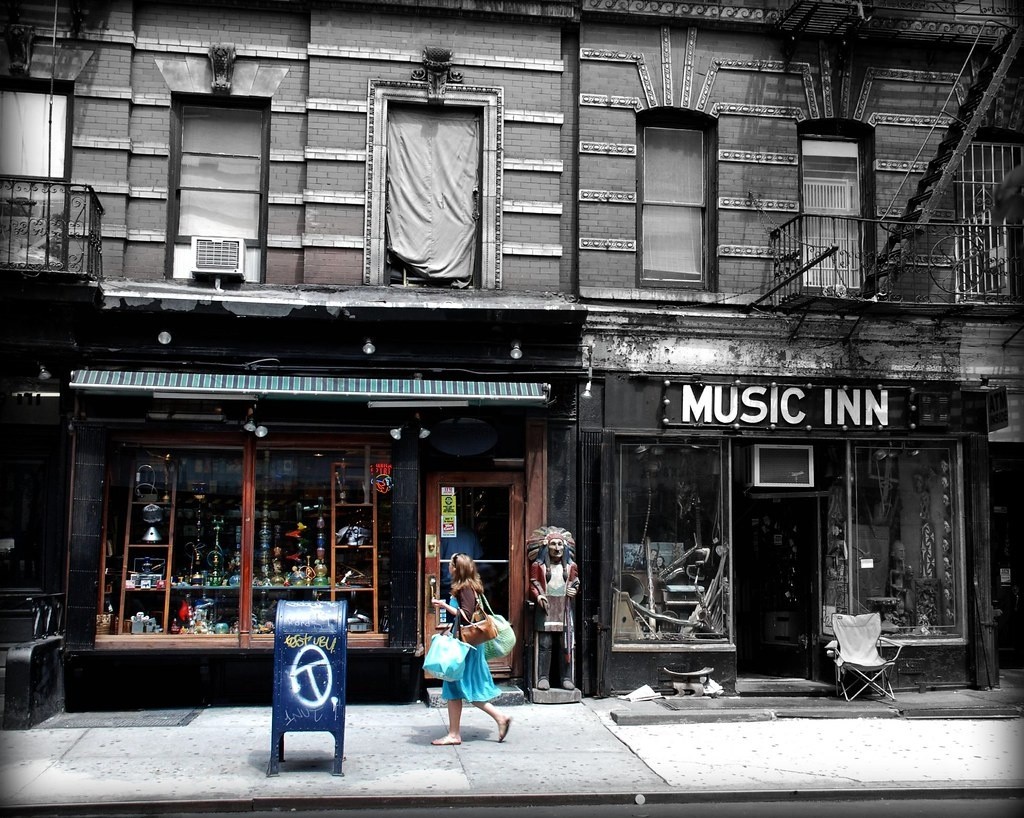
[191,236,246,283]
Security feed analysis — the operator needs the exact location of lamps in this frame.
[390,428,401,439]
[510,342,522,359]
[363,340,375,354]
[580,381,592,398]
[419,426,431,438]
[38,365,51,380]
[244,419,268,438]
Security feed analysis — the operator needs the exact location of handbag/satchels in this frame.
[459,612,497,645]
[422,607,477,682]
[484,615,516,660]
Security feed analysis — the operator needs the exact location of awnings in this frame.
[69,372,552,404]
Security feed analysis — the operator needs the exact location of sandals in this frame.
[499,719,510,742]
[432,738,461,745]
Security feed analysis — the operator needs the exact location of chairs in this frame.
[825,612,903,703]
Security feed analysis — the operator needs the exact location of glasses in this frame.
[453,552,466,568]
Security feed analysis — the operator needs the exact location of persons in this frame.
[440,515,481,624]
[431,554,514,745]
[890,541,914,632]
[625,548,665,569]
[527,527,582,689]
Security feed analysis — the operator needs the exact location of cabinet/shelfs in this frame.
[118,453,179,634]
[331,461,380,633]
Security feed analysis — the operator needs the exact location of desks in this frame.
[171,581,331,609]
